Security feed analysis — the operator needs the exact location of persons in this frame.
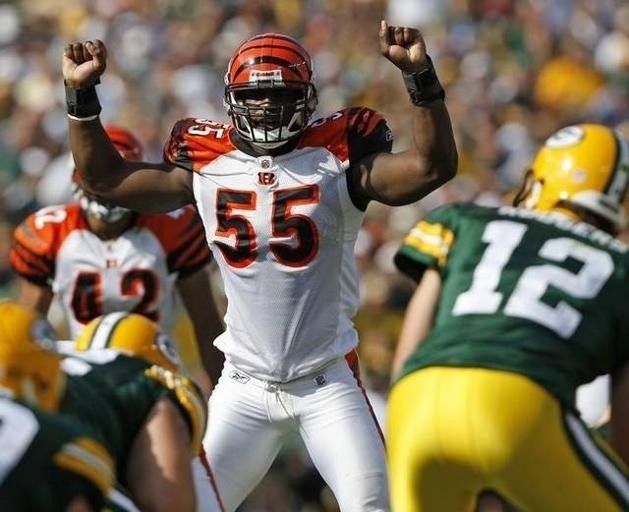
[385,123,628,511]
[52,310,209,511]
[63,21,459,511]
[0,0,627,303]
[9,127,225,438]
[0,299,140,511]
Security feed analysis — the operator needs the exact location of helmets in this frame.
[524,122,628,223]
[68,128,147,222]
[73,309,182,376]
[222,30,320,145]
[0,297,61,414]
